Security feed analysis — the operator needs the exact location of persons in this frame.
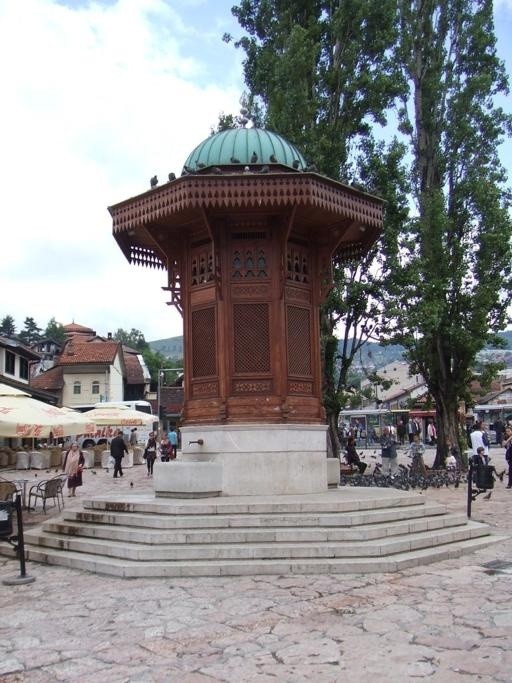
[158,431,173,461]
[110,430,129,476]
[167,427,177,458]
[61,441,85,497]
[337,416,512,489]
[143,431,158,476]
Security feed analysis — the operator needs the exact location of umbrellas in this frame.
[0,381,160,450]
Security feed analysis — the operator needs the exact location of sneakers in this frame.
[68,494,77,498]
[112,472,125,479]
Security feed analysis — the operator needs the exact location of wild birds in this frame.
[250,152,258,163]
[293,160,299,169]
[483,491,493,499]
[150,175,158,186]
[230,157,239,163]
[343,464,474,491]
[169,172,176,181]
[195,160,206,168]
[269,153,279,163]
[184,165,194,173]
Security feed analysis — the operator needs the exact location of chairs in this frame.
[0,444,145,470]
[0,474,68,516]
